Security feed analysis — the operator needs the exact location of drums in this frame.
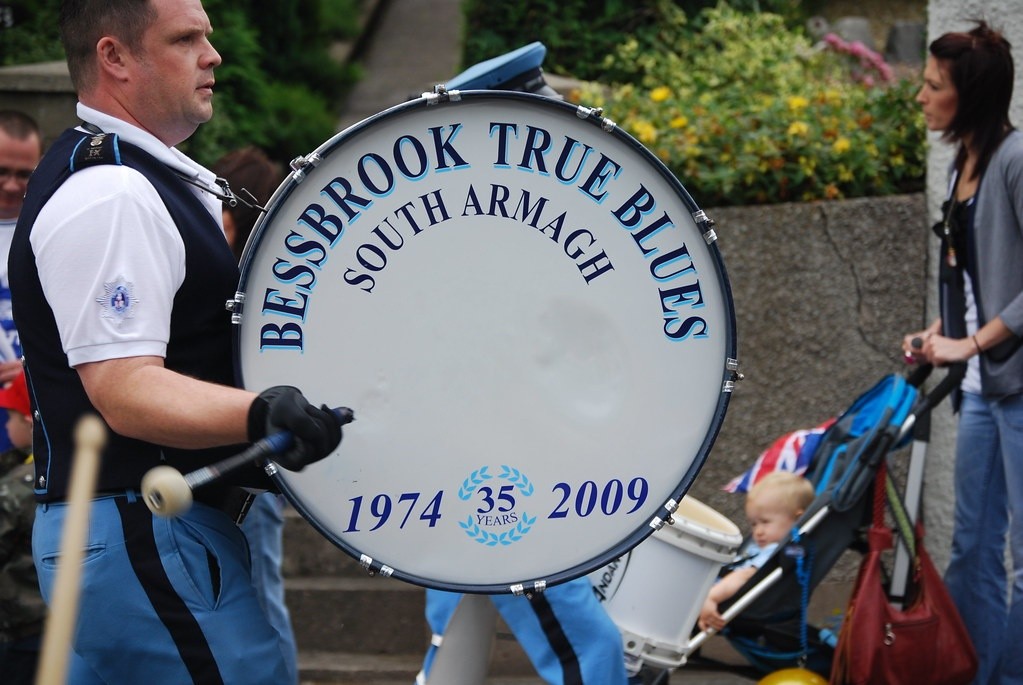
[238,86,745,601]
[587,488,747,674]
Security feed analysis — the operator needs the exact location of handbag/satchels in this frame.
[829,461,979,685]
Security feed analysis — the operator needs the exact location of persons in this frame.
[0,0,345,685]
[416,42,625,685]
[699,470,816,636]
[916,18,1023,685]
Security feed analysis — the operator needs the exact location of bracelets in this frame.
[973,335,982,354]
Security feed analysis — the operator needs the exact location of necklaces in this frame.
[943,157,974,266]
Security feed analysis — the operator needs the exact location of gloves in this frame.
[247,384,343,473]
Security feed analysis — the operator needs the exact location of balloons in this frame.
[758,668,827,685]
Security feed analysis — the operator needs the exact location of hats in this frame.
[442,40,563,100]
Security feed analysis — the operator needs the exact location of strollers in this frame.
[419,355,966,685]
